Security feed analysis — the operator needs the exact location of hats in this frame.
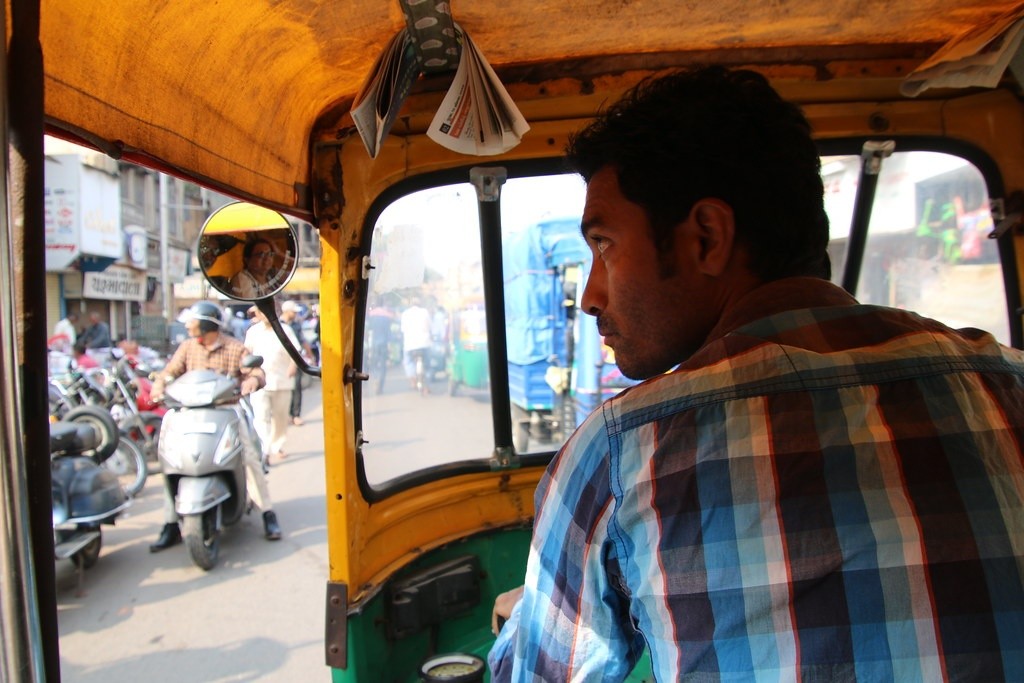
[281,299,303,314]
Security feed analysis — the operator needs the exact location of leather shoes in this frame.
[147,523,182,553]
[262,511,281,540]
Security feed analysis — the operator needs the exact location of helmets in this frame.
[181,297,223,328]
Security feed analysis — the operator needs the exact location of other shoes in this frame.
[262,454,270,472]
[271,444,286,458]
[289,414,302,424]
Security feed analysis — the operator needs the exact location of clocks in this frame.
[129,234,146,263]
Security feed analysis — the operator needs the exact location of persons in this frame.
[151,299,281,551]
[243,308,300,467]
[485,62,1023,683]
[50,295,452,426]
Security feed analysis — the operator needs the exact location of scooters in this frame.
[49,418,130,562]
[150,354,265,572]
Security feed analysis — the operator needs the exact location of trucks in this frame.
[502,217,677,448]
